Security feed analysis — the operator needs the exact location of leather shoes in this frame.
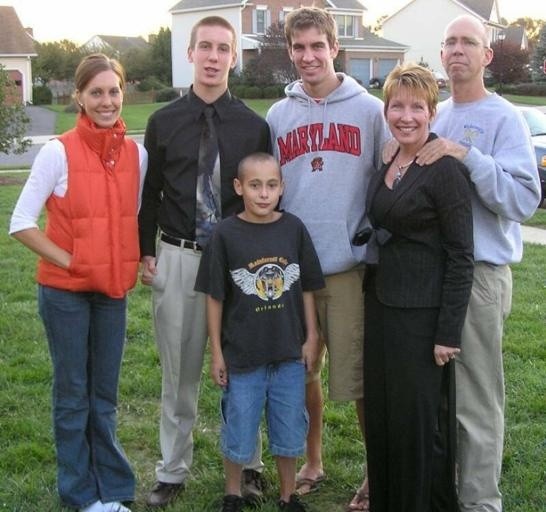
[145,479,186,507]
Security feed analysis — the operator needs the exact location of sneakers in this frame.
[240,469,267,505]
[279,493,306,512]
[222,494,241,512]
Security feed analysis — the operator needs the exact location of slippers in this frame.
[347,488,372,512]
[293,462,328,496]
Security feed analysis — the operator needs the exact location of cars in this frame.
[516,107,546,210]
[432,71,447,90]
[369,77,385,88]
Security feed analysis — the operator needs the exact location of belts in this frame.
[157,231,205,252]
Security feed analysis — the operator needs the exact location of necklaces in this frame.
[388,154,415,189]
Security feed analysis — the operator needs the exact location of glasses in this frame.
[440,39,489,49]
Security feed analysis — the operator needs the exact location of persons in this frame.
[7,49,149,512]
[362,58,476,512]
[264,5,394,511]
[139,15,273,509]
[194,151,327,511]
[416,13,543,511]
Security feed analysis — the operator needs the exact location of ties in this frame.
[193,104,223,251]
